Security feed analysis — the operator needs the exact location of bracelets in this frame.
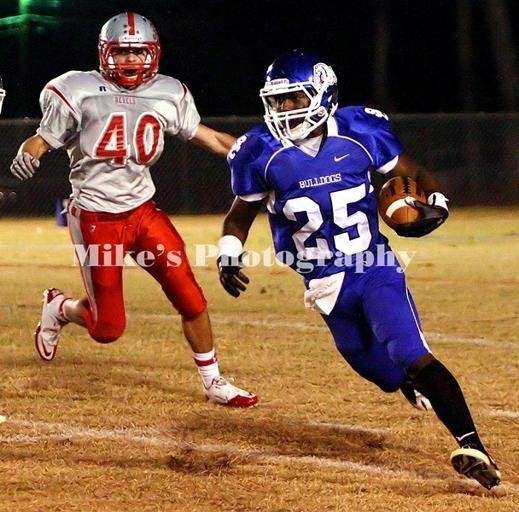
[215,235,243,259]
[426,191,450,211]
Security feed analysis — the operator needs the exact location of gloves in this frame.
[10,151,40,181]
[396,197,448,237]
[216,252,249,297]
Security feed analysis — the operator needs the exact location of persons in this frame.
[216,48,501,489]
[9,13,260,409]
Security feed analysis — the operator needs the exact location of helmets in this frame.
[98,13,161,88]
[259,50,338,143]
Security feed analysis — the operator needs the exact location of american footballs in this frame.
[378,175,426,229]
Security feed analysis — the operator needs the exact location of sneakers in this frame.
[450,444,501,490]
[34,288,64,361]
[203,376,259,409]
[400,377,432,411]
